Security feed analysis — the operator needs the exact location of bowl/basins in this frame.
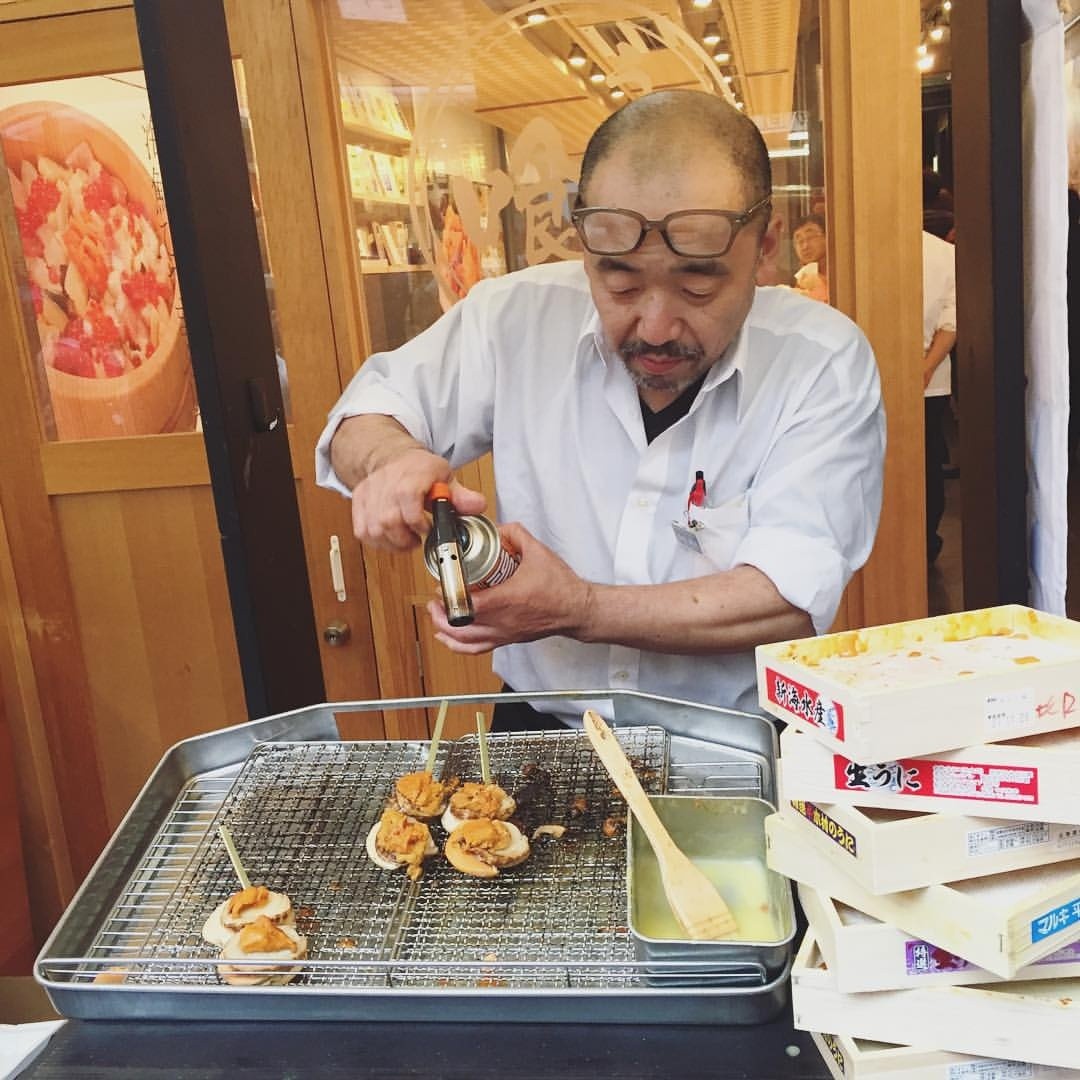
[626,793,796,990]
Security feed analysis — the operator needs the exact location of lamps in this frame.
[527,0,546,21]
[568,44,586,66]
[589,62,606,81]
[787,112,808,140]
[723,69,732,81]
[610,87,625,98]
[713,40,731,62]
[703,22,721,44]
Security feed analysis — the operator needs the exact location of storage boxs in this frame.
[786,927,1080,1069]
[808,1032,1080,1080]
[625,790,793,979]
[753,602,1080,764]
[762,779,1080,896]
[764,813,1080,980]
[794,880,1080,995]
[776,716,1080,826]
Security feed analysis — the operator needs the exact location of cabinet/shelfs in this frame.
[343,119,434,273]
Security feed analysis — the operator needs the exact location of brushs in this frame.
[584,707,736,942]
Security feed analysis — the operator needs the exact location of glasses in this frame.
[568,195,770,260]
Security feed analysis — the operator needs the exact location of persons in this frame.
[313,92,887,756]
[793,168,957,570]
